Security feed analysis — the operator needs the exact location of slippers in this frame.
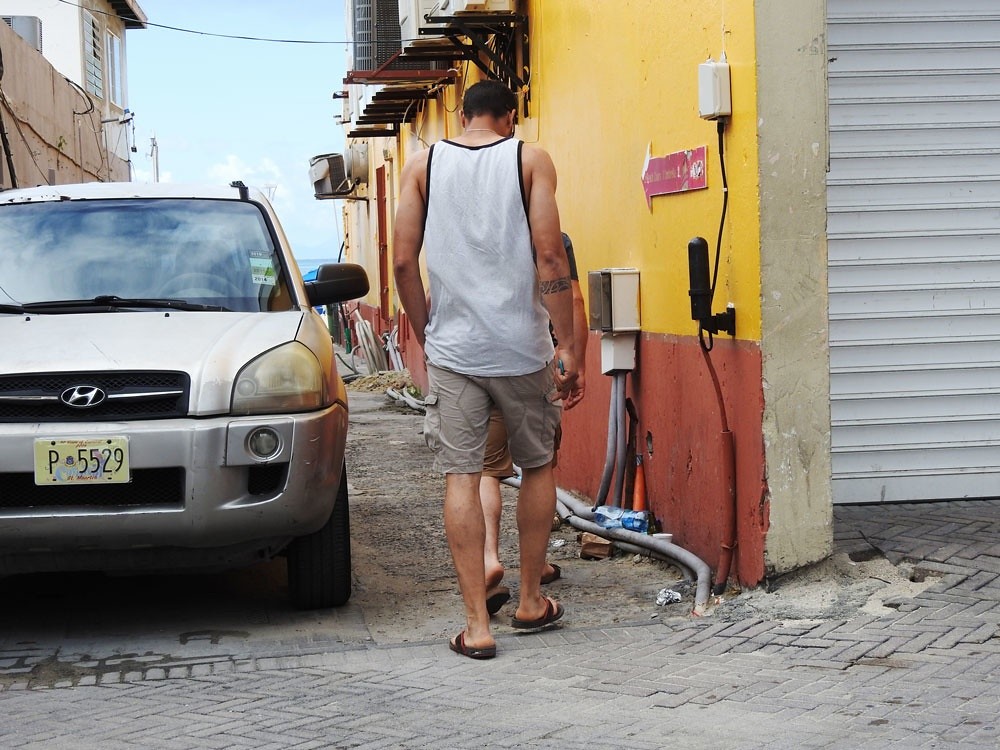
[511,597,564,628]
[540,563,559,584]
[449,631,496,658]
[487,586,510,613]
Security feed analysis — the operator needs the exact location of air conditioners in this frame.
[0,15,42,54]
[345,0,515,131]
[310,154,350,194]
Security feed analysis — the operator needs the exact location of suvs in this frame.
[2,181,370,612]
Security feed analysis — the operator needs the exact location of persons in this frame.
[393,80,577,659]
[471,232,588,615]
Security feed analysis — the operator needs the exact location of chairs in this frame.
[172,239,254,297]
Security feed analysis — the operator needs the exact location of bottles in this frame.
[593,505,659,534]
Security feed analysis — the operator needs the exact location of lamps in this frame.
[102,112,133,125]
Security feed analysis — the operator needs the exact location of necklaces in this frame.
[466,128,497,133]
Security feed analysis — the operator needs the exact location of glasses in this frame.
[509,105,516,138]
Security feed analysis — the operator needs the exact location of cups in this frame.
[653,533,673,542]
